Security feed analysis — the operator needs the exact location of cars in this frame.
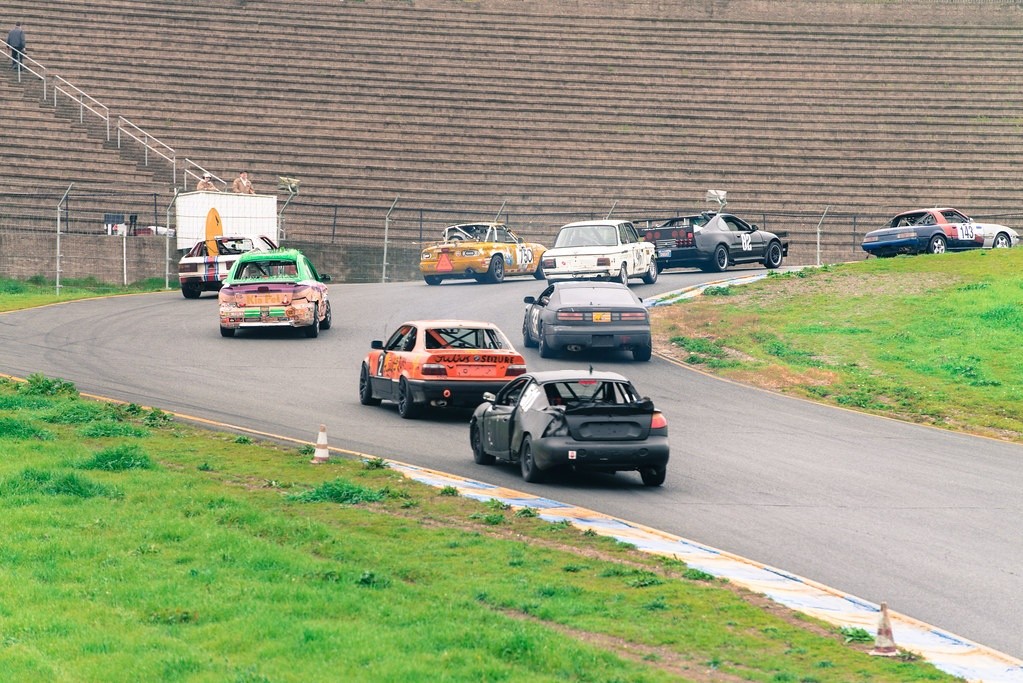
[177,239,261,299]
[521,280,652,362]
[217,247,332,338]
[469,369,671,488]
[358,319,529,420]
[540,218,658,288]
[419,222,551,286]
[860,206,1020,259]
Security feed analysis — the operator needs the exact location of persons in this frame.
[197,172,221,193]
[233,170,256,195]
[7,21,27,71]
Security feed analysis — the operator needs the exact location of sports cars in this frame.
[630,209,789,273]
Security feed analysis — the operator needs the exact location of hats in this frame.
[240,171,247,174]
[203,173,211,177]
